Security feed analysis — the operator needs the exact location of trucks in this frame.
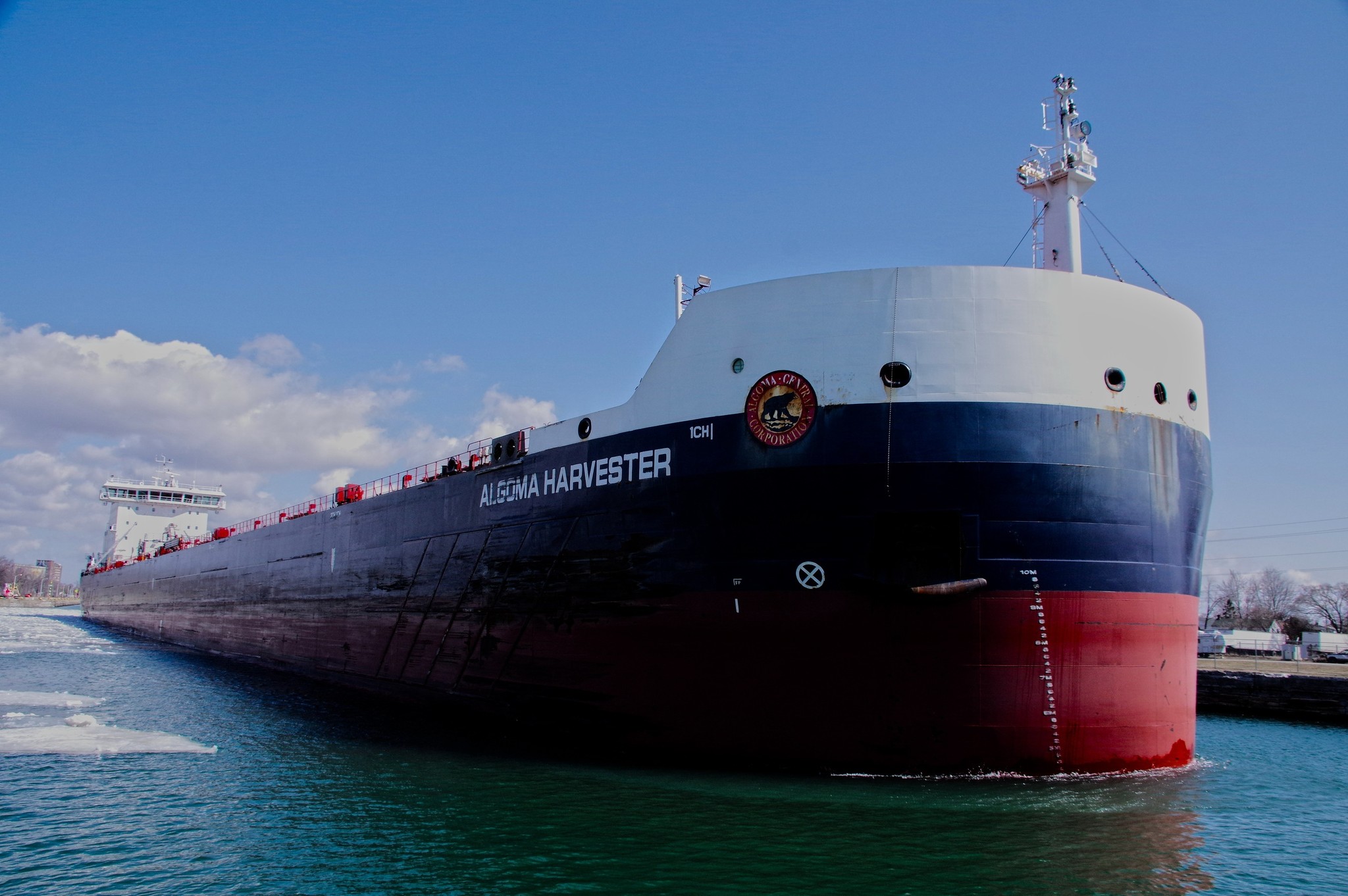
[1198,630,1226,658]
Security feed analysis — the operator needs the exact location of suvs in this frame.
[1326,649,1348,663]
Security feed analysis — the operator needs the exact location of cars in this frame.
[59,592,66,594]
[1311,654,1326,663]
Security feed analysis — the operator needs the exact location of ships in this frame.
[79,71,1215,783]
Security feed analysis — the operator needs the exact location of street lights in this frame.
[40,578,49,596]
[12,574,22,596]
